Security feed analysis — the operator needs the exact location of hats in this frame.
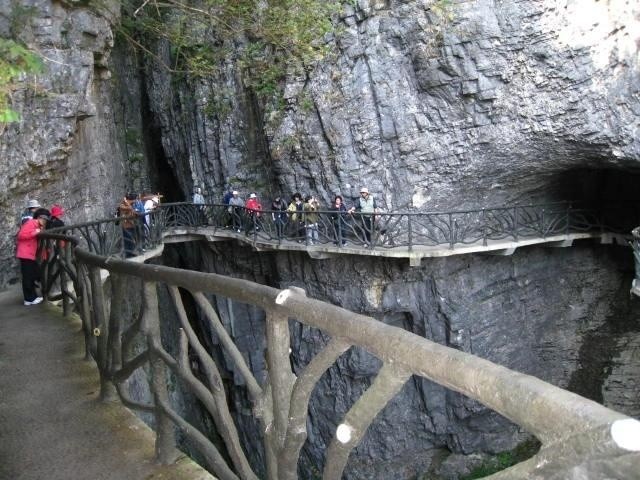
[232,191,238,195]
[249,194,257,198]
[50,206,64,217]
[39,214,52,223]
[27,200,40,209]
[360,188,369,195]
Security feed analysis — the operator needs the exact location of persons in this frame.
[193,187,208,225]
[20,198,43,224]
[222,187,234,226]
[15,208,51,305]
[227,190,244,232]
[48,204,66,230]
[131,193,147,253]
[330,195,348,247]
[119,192,138,258]
[143,192,160,245]
[303,195,322,246]
[286,192,305,242]
[271,191,286,236]
[244,193,262,231]
[347,187,377,248]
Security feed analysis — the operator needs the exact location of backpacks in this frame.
[113,206,121,225]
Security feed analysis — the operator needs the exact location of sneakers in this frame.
[23,297,44,306]
